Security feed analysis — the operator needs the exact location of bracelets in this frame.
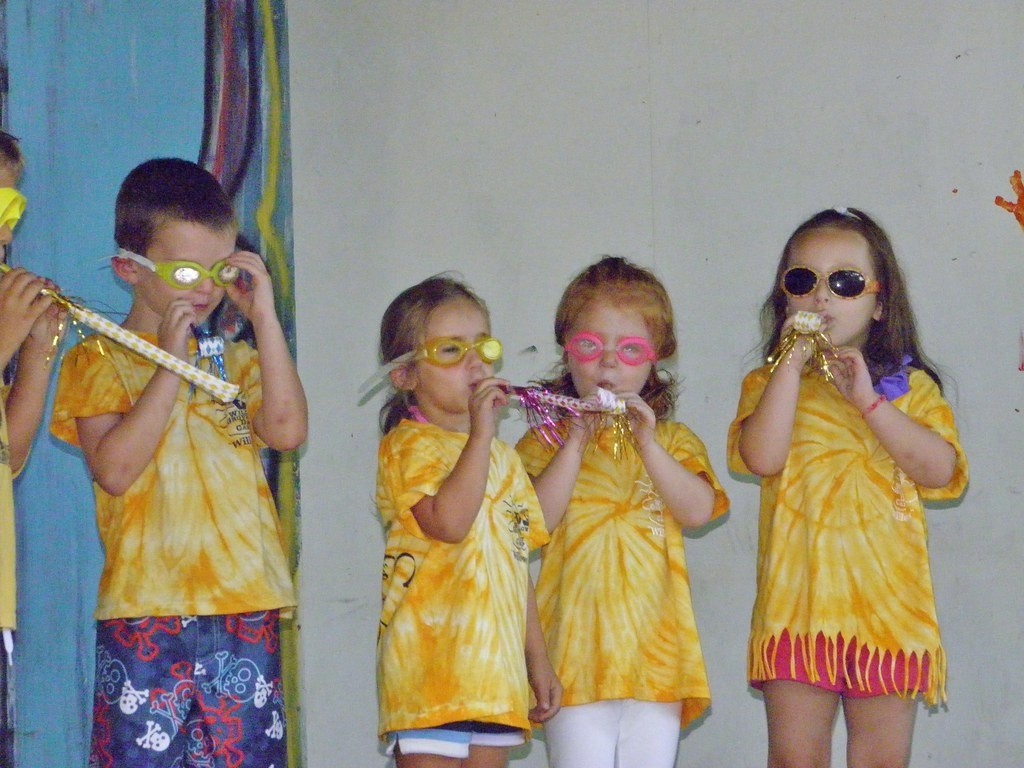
[860,391,888,419]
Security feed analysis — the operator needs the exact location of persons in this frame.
[726,205,970,768]
[513,258,728,768]
[50,160,309,768]
[0,129,66,768]
[372,277,565,767]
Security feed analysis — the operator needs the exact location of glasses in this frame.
[118,247,240,291]
[563,334,657,366]
[778,265,882,300]
[0,188,28,231]
[408,338,504,367]
